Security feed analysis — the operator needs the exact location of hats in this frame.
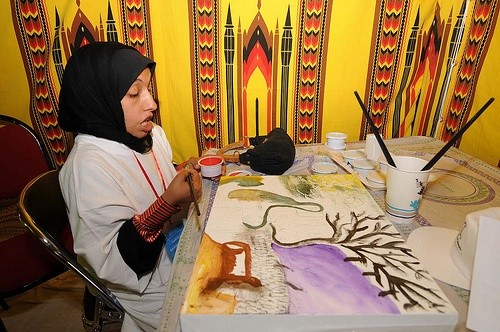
[406,206,500,292]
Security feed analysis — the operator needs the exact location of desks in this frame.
[156,136,500,332]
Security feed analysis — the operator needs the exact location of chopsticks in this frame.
[354,90,495,171]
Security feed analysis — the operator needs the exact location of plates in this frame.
[325,142,347,151]
[340,149,395,190]
[311,162,338,175]
[228,171,252,176]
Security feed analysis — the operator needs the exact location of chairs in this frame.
[0,114,126,332]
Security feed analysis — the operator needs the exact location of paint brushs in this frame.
[423,97,496,170]
[354,90,396,167]
[184,163,201,216]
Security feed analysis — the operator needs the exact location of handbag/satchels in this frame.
[217,128,295,176]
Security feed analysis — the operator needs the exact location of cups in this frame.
[366,133,383,161]
[385,156,432,224]
[326,132,348,148]
[198,155,224,177]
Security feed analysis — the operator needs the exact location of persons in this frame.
[57,40,203,332]
